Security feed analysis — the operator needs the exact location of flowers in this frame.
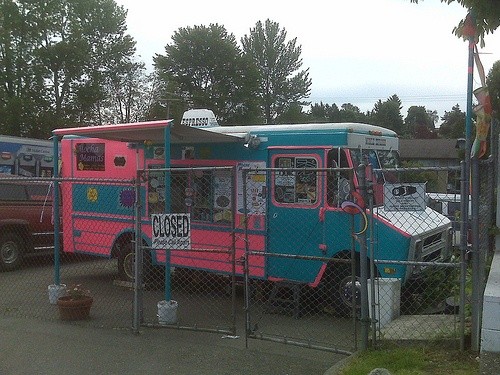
[59,277,91,301]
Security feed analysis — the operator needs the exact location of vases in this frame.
[57,295,93,321]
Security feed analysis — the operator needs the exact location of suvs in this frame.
[0,172,62,270]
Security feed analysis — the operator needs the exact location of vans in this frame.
[424,193,476,246]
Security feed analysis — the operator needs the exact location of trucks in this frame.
[0,135,62,178]
[62,109,453,321]
